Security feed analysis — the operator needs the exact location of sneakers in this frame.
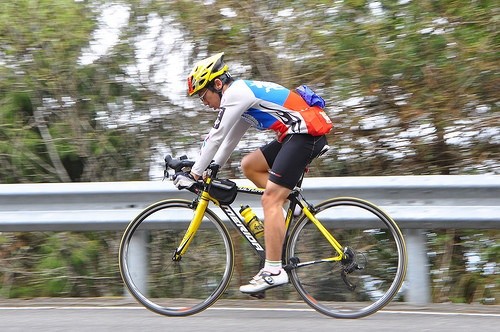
[239,267,288,294]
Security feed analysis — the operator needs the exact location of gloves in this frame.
[173,175,199,190]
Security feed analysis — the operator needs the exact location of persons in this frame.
[172,51,334,293]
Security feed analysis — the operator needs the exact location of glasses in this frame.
[199,88,209,101]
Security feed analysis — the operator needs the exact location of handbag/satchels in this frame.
[296,84,325,109]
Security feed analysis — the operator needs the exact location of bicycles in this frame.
[118,155,408,317]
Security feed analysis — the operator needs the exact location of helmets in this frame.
[187,51,229,97]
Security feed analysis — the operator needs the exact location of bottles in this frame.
[238,203,264,238]
